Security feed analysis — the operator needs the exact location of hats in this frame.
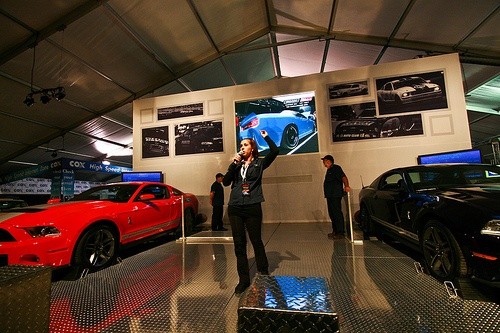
[321,155,334,163]
[216,173,225,177]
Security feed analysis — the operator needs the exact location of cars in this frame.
[236,97,317,153]
[377,77,443,107]
[329,83,368,98]
[359,162,500,287]
[0,179,200,275]
[334,107,424,142]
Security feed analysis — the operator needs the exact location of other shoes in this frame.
[328,232,345,238]
[212,227,228,231]
[235,279,250,293]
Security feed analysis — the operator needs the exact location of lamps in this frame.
[45,149,65,158]
[24,87,66,107]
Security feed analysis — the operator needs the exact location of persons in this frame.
[223,130,279,295]
[209,173,228,231]
[320,154,351,239]
